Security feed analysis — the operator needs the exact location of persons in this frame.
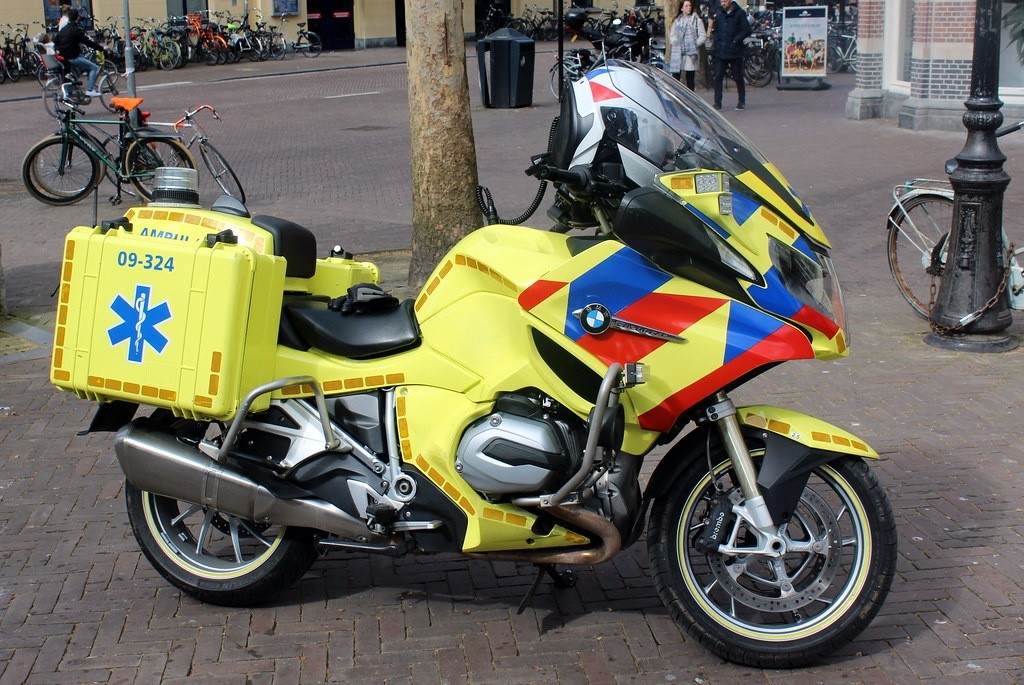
[47,0,61,20]
[669,0,708,94]
[653,74,681,121]
[699,0,753,111]
[52,0,106,97]
[74,0,89,26]
[785,32,825,70]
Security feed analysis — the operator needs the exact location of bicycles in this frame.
[549,1,858,101]
[21,91,246,207]
[474,0,665,43]
[0,8,322,84]
[886,118,1024,325]
[37,47,127,119]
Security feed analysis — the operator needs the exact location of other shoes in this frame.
[86,89,102,96]
[734,103,746,111]
[712,103,721,110]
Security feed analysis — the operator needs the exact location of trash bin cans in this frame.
[476,26,536,108]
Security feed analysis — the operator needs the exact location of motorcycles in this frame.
[48,55,899,670]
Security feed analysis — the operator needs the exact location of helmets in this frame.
[555,66,665,169]
